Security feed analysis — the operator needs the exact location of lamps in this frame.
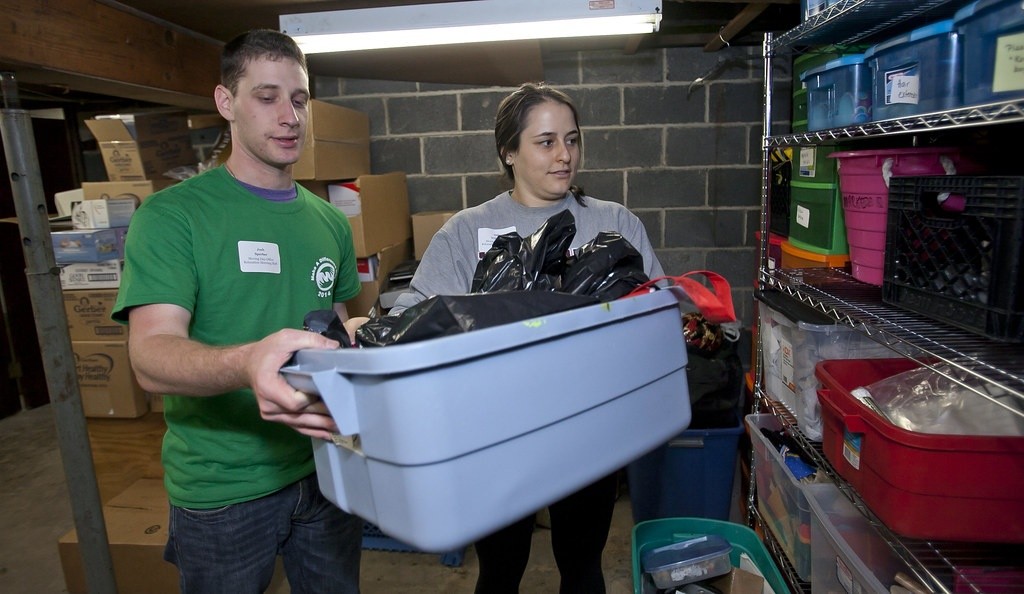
[277,0,663,53]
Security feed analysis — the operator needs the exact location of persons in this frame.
[389,84,668,594]
[113,32,370,594]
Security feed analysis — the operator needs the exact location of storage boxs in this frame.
[46,0,1024,594]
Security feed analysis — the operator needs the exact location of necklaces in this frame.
[224,160,236,178]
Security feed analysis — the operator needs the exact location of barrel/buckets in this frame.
[826,146,980,284]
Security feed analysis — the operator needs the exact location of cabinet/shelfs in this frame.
[746,0,1024,594]
